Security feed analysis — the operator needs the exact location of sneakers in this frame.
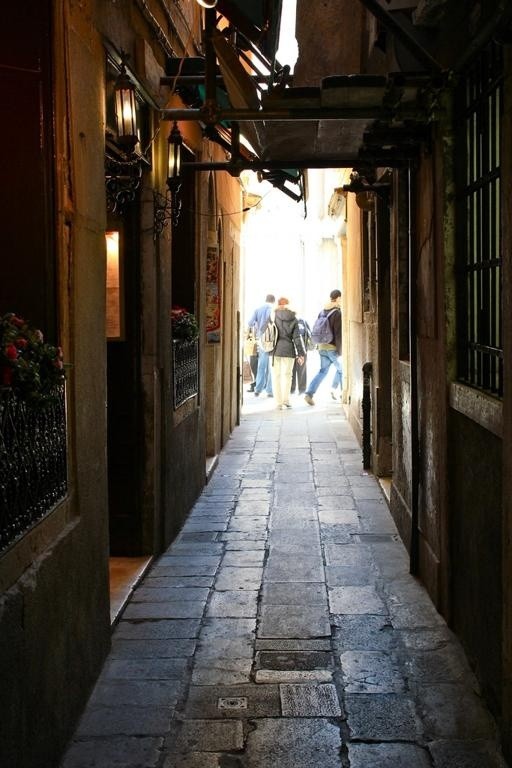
[331,389,337,400]
[277,401,291,410]
[305,394,315,406]
[246,387,273,398]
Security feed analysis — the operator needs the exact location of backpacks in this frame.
[311,309,337,345]
[259,310,278,352]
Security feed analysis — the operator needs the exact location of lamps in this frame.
[105,44,150,216]
[153,114,185,239]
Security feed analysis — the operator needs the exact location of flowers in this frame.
[170,305,201,343]
[0,312,74,413]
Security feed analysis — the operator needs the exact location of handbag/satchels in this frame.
[245,325,256,357]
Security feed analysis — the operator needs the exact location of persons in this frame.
[245,290,342,405]
